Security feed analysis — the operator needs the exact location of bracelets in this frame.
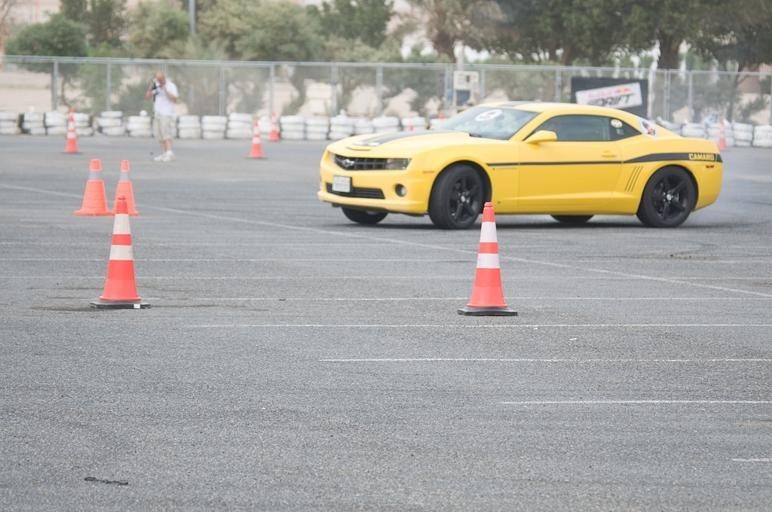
[152,88,160,96]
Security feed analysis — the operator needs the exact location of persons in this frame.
[145,71,179,162]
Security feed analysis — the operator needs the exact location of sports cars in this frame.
[318,99,724,229]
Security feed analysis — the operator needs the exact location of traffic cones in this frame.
[244,123,267,159]
[71,159,115,220]
[61,114,84,156]
[89,193,153,312]
[112,160,139,218]
[268,112,279,142]
[714,113,729,151]
[454,202,520,319]
